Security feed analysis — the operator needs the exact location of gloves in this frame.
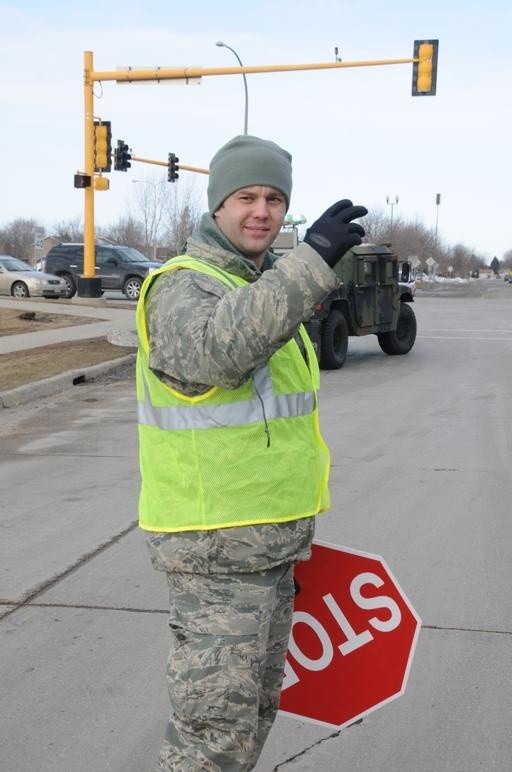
[303,199,368,268]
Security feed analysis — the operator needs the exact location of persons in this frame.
[135,135,368,771]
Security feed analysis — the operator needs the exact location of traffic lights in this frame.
[73,175,92,189]
[168,153,179,182]
[119,144,132,172]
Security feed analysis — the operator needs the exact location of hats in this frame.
[208,135,292,217]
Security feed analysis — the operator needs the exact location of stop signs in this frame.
[275,538,421,731]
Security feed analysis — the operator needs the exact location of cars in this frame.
[0,255,68,300]
[503,275,512,283]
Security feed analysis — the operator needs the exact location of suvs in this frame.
[46,243,166,300]
[301,242,416,369]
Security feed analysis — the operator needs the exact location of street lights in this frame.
[386,195,398,236]
[434,194,440,242]
[216,41,249,136]
